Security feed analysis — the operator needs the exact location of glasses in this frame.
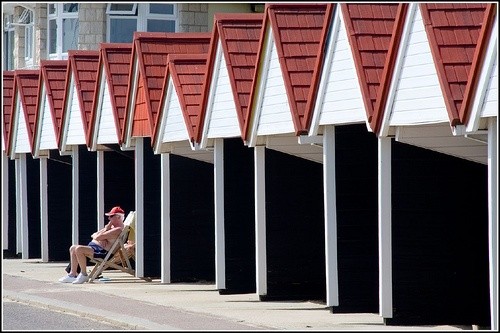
[108,215,118,218]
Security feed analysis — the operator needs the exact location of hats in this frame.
[105,206,125,218]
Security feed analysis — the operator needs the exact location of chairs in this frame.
[83,210,136,283]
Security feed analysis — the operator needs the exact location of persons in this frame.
[63,206,125,284]
[65,240,135,278]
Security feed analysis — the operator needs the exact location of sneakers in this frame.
[59,274,77,283]
[72,273,89,285]
